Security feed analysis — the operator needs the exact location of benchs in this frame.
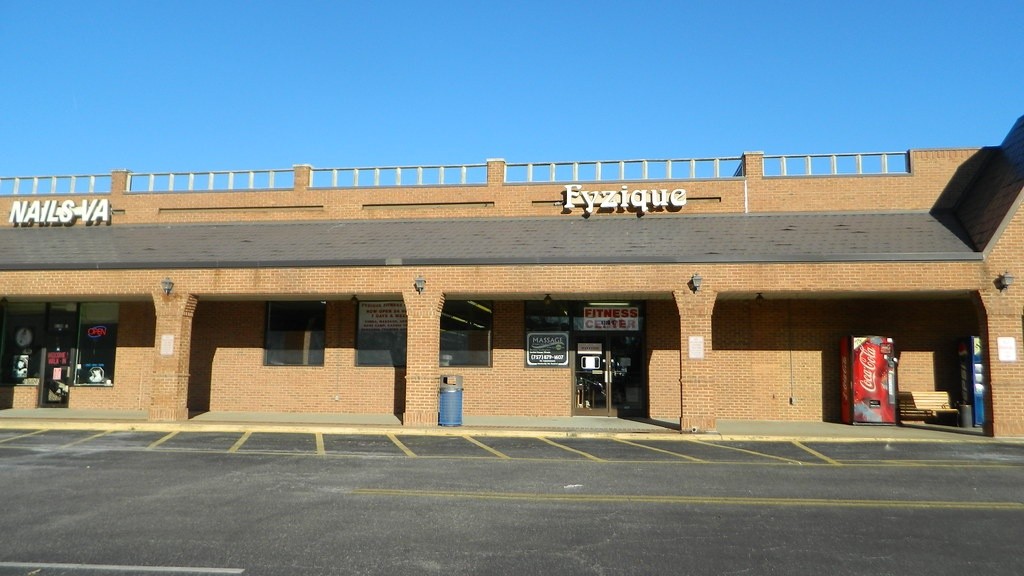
[911,390,959,421]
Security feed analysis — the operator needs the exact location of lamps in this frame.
[161,277,174,294]
[415,274,427,292]
[999,270,1015,287]
[692,273,702,288]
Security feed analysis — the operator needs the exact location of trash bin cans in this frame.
[439,375,465,427]
[958,404,972,428]
